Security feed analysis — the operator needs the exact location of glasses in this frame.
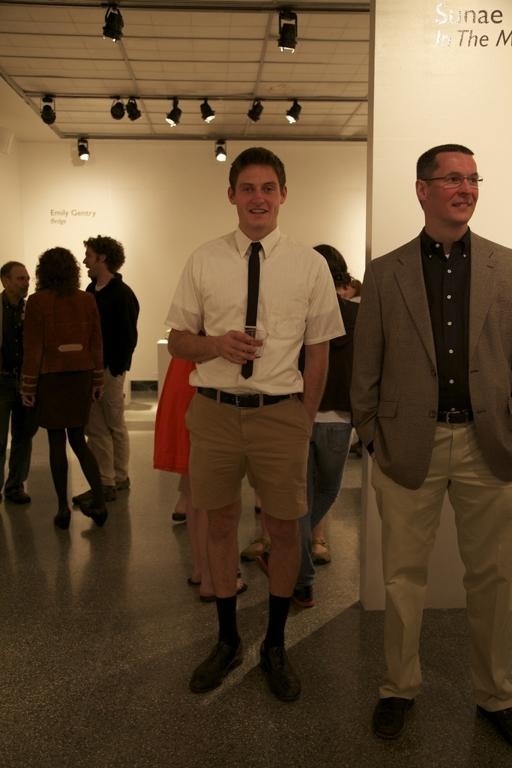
[422,172,485,190]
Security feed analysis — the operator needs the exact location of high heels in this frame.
[55,511,71,530]
[81,505,108,526]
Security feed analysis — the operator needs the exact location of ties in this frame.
[240,241,263,380]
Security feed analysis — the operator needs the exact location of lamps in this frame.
[100,0,303,53]
[30,92,306,128]
[73,135,234,161]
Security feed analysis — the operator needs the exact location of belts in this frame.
[437,406,475,426]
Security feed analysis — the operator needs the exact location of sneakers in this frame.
[71,486,117,503]
[116,477,130,490]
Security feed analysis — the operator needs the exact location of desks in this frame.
[157,331,181,392]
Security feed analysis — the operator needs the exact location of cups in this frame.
[243,330,269,358]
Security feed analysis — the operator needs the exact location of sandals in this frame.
[171,510,333,609]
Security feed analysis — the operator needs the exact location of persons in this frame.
[17,246,109,529]
[163,147,350,701]
[0,260,32,505]
[153,244,362,608]
[69,236,140,507]
[345,146,512,746]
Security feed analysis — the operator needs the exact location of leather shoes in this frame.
[370,696,416,740]
[475,703,512,745]
[196,385,300,409]
[259,638,302,704]
[188,636,244,695]
[6,491,31,505]
